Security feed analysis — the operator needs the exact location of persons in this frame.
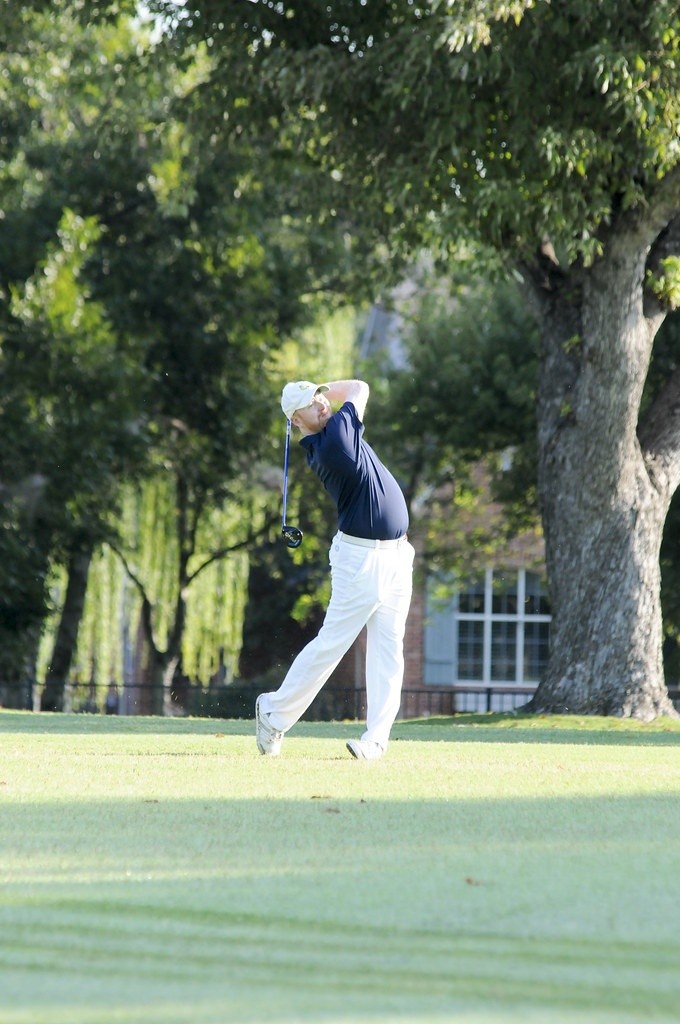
[256,379,415,758]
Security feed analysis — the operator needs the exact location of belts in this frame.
[336,530,408,550]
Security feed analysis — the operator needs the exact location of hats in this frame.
[281,380,330,420]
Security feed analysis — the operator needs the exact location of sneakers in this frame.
[346,739,383,761]
[255,693,285,756]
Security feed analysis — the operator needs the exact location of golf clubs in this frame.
[282,418,303,547]
